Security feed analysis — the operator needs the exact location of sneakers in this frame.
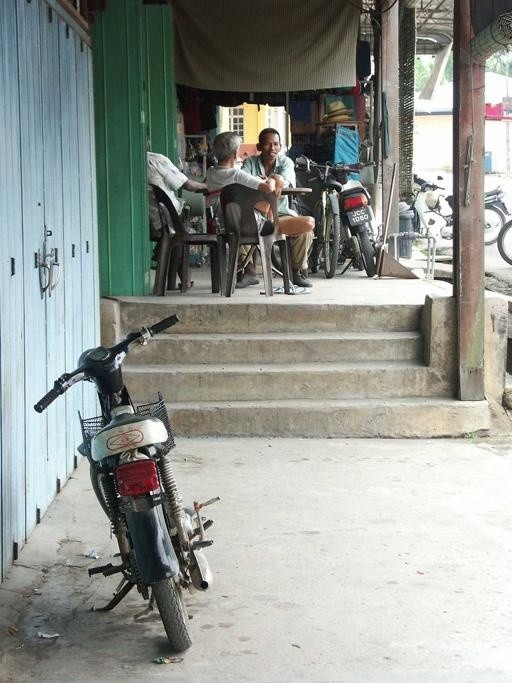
[235,275,260,289]
[292,271,313,286]
[178,280,194,290]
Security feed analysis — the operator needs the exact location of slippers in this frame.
[259,285,285,294]
[287,285,312,294]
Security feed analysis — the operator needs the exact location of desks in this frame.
[260,187,312,294]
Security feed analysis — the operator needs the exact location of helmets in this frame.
[424,190,440,209]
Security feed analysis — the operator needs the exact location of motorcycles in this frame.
[411,173,508,248]
[308,162,381,278]
[34,316,219,652]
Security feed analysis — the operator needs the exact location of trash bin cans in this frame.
[399,201,415,259]
[484,151,492,174]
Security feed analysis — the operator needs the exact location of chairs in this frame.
[144,184,294,295]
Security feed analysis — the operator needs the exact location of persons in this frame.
[147,132,208,293]
[194,131,315,247]
[240,128,316,290]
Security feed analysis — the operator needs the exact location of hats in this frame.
[321,100,355,122]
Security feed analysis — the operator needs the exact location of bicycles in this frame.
[267,166,317,278]
[296,154,361,278]
